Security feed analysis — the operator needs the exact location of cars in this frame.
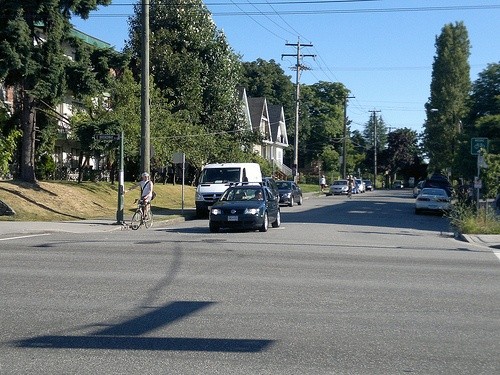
[392,180,404,190]
[354,179,366,193]
[262,178,280,203]
[363,180,373,191]
[413,182,424,197]
[329,179,350,196]
[276,180,303,208]
[207,186,281,233]
[414,188,451,216]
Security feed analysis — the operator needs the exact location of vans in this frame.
[194,161,263,218]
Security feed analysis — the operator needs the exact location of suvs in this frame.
[424,174,452,198]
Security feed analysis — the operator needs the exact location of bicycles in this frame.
[130,198,153,230]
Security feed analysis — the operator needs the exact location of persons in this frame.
[125,171,153,219]
[346,174,356,192]
[320,174,326,191]
